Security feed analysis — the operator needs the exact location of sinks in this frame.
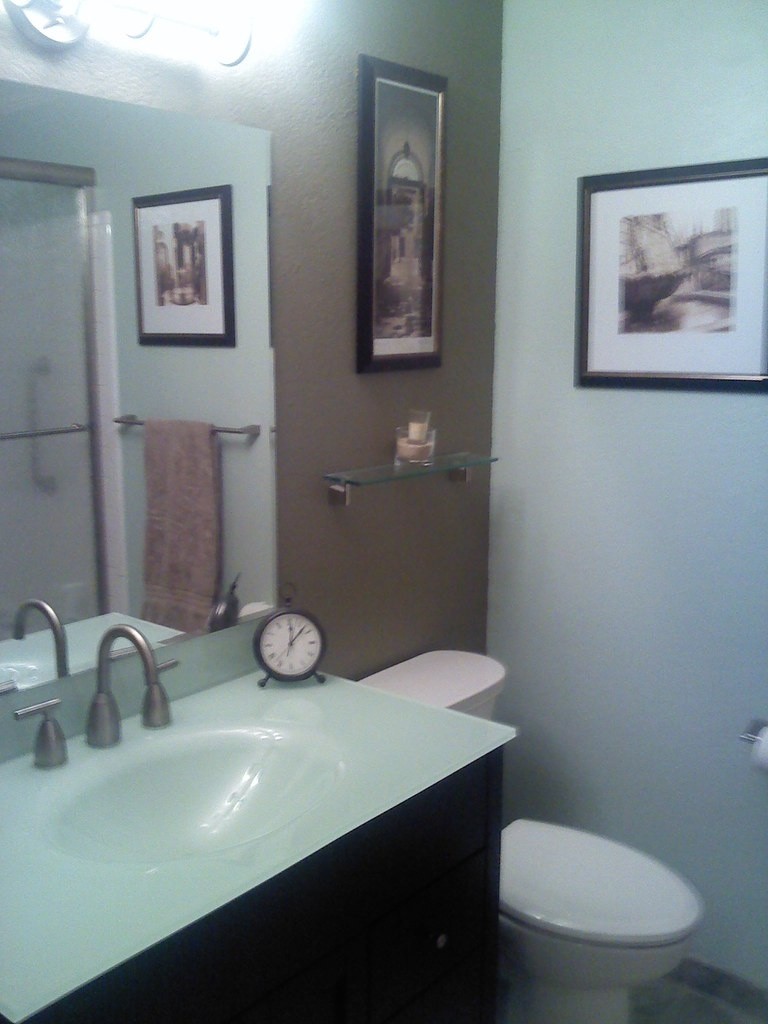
[0,662,40,687]
[38,726,341,866]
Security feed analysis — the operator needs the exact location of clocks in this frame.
[208,574,241,625]
[253,580,326,690]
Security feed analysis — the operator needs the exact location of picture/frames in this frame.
[130,183,237,346]
[356,53,449,374]
[573,156,768,392]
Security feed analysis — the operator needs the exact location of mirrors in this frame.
[0,77,278,700]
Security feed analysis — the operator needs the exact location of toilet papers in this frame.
[751,725,768,772]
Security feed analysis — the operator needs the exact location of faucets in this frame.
[11,598,70,678]
[86,623,170,748]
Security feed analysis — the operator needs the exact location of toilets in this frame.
[356,647,708,1024]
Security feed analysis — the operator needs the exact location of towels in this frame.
[142,420,222,636]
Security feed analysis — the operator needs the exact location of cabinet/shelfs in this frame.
[24,742,506,1023]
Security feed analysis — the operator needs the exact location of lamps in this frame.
[12,0,256,67]
